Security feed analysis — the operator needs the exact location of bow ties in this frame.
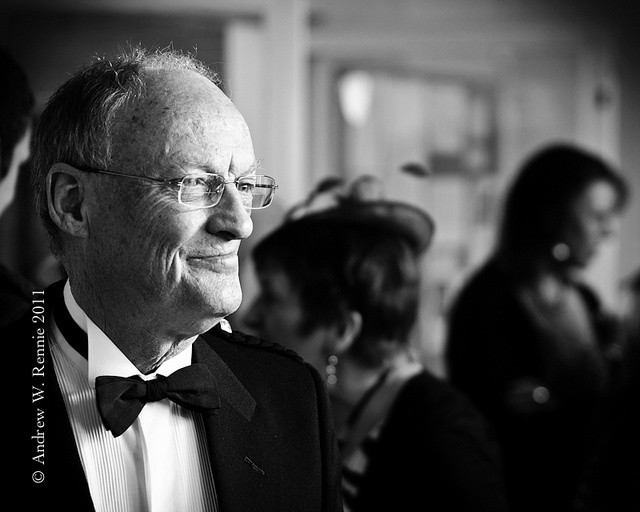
[95,365,216,435]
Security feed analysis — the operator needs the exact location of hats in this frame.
[251,177,432,257]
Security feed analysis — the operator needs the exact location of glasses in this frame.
[90,170,275,210]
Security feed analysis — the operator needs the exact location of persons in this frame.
[0,52,342,512]
[242,176,509,512]
[443,143,639,512]
